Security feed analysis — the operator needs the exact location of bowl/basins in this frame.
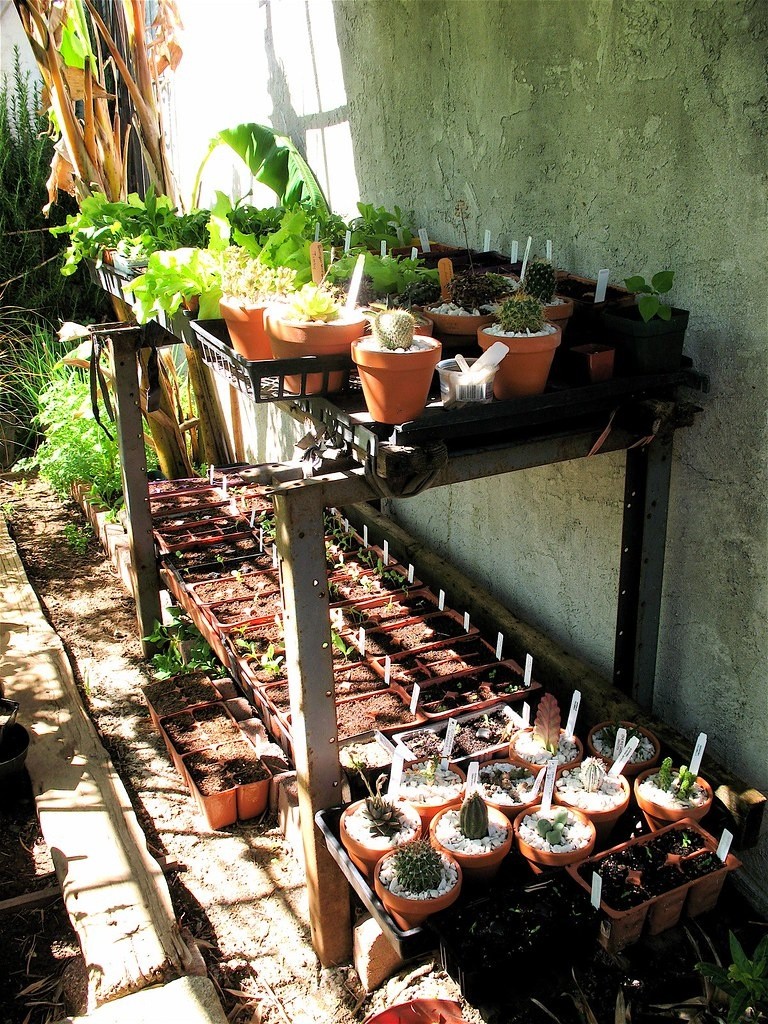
[435,357,500,406]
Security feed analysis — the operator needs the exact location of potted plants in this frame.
[10,186,742,955]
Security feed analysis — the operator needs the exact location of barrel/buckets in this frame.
[0,722,30,796]
